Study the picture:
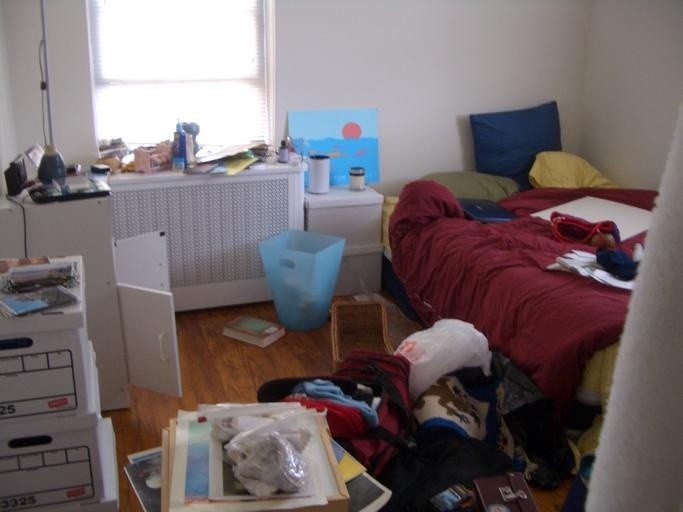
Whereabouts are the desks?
[108,162,306,314]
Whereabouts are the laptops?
[457,197,515,223]
[27,177,112,204]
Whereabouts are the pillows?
[529,152,618,188]
[422,171,520,203]
[469,99,562,192]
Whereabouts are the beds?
[383,188,660,431]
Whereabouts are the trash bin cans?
[260,230,346,332]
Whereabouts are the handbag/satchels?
[473,473,539,512]
[551,211,621,244]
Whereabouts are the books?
[0,285,79,315]
[223,314,287,349]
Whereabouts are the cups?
[308,155,330,193]
[75,159,92,180]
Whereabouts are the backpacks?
[258,349,413,480]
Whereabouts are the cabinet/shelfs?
[0,177,182,412]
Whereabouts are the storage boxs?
[0,256,120,512]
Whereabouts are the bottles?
[350,167,365,190]
[278,139,289,163]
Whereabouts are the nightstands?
[304,184,384,297]
